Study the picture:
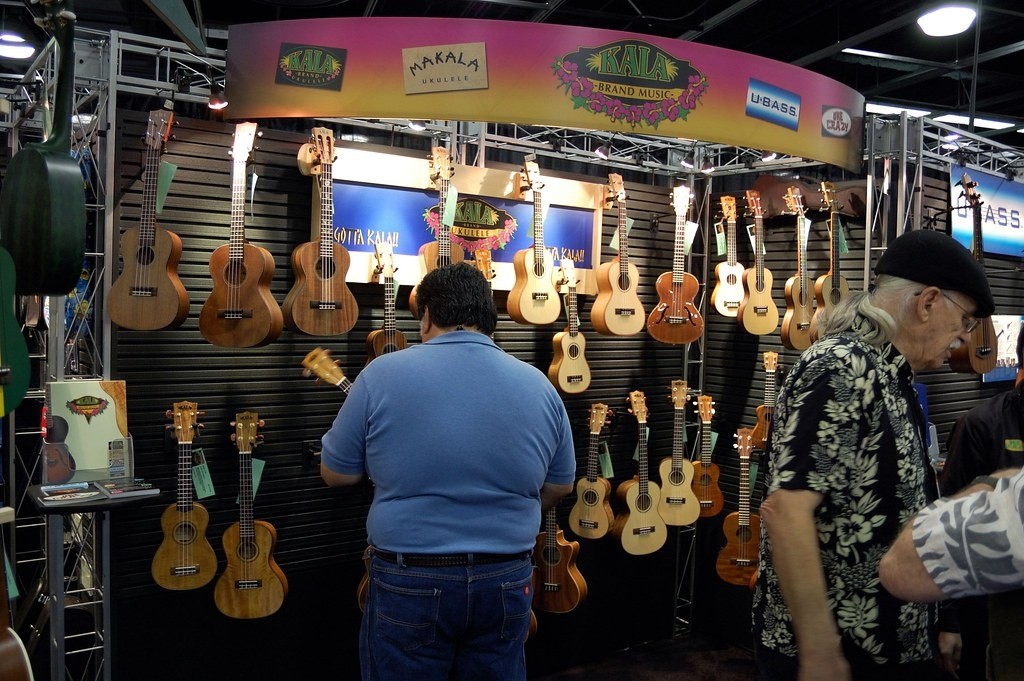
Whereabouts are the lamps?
[174,68,230,109]
[408,120,427,132]
[761,150,776,162]
[701,145,753,174]
[632,142,697,169]
[553,131,617,160]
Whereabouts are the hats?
[875,229,996,319]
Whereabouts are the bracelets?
[971,475,999,489]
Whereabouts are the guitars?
[548,258,591,394]
[469,248,496,344]
[409,145,464,320]
[647,184,704,345]
[365,242,408,366]
[708,179,850,351]
[214,409,288,619]
[507,161,561,326]
[199,121,283,348]
[301,347,354,394]
[591,173,646,337]
[150,399,217,591]
[714,426,760,586]
[532,503,588,613]
[281,126,359,336]
[107,109,191,331]
[947,172,998,374]
[569,377,724,556]
[750,352,779,451]
[0,0,87,681]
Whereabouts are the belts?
[373,548,530,569]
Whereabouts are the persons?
[937,329,1024,679]
[879,465,1024,681]
[321,260,576,681]
[751,229,995,681]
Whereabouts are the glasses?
[914,289,981,333]
